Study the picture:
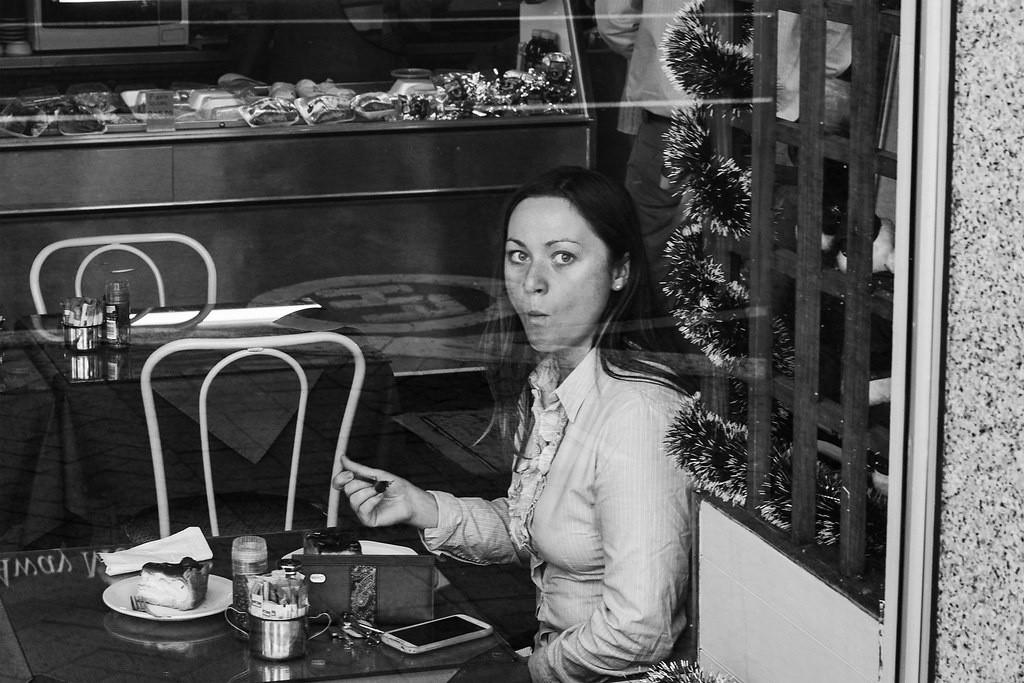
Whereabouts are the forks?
[342,468,395,494]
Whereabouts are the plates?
[237,87,445,128]
[103,574,232,622]
[0,17,28,41]
[0,92,120,138]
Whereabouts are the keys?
[329,614,383,649]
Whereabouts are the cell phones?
[381,614,494,654]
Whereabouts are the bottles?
[103,278,131,351]
[516,29,558,72]
[231,536,268,622]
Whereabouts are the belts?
[641,109,686,126]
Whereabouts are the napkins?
[98,526,214,576]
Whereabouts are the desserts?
[303,533,363,555]
[135,557,209,610]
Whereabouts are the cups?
[225,605,332,660]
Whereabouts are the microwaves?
[25,0,189,51]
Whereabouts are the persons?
[594,0,706,313]
[332,166,696,682]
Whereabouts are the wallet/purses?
[291,554,436,622]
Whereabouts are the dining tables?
[0,524,526,683]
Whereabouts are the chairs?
[139,330,366,539]
[29,231,217,315]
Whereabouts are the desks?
[14,299,410,549]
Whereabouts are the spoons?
[338,612,385,643]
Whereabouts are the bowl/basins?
[390,67,431,84]
[61,320,105,351]
[4,41,32,56]
